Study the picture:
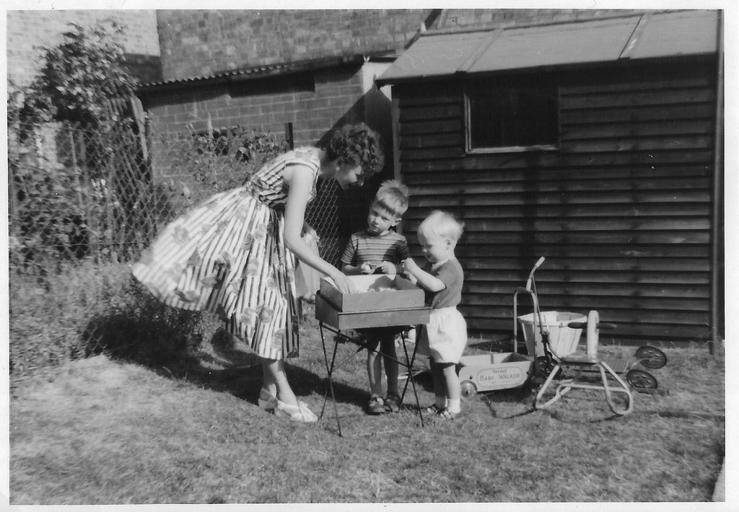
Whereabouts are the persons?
[342,179,414,415]
[129,122,384,425]
[399,209,468,421]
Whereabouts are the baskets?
[518,311,587,356]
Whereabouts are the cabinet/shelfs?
[317,321,425,436]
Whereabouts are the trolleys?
[458,256,563,402]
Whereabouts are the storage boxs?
[320,274,425,311]
[314,290,432,330]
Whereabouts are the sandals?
[422,405,444,416]
[435,413,460,425]
[384,397,398,410]
[368,396,386,414]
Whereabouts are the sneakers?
[258,390,279,411]
[271,401,316,423]
